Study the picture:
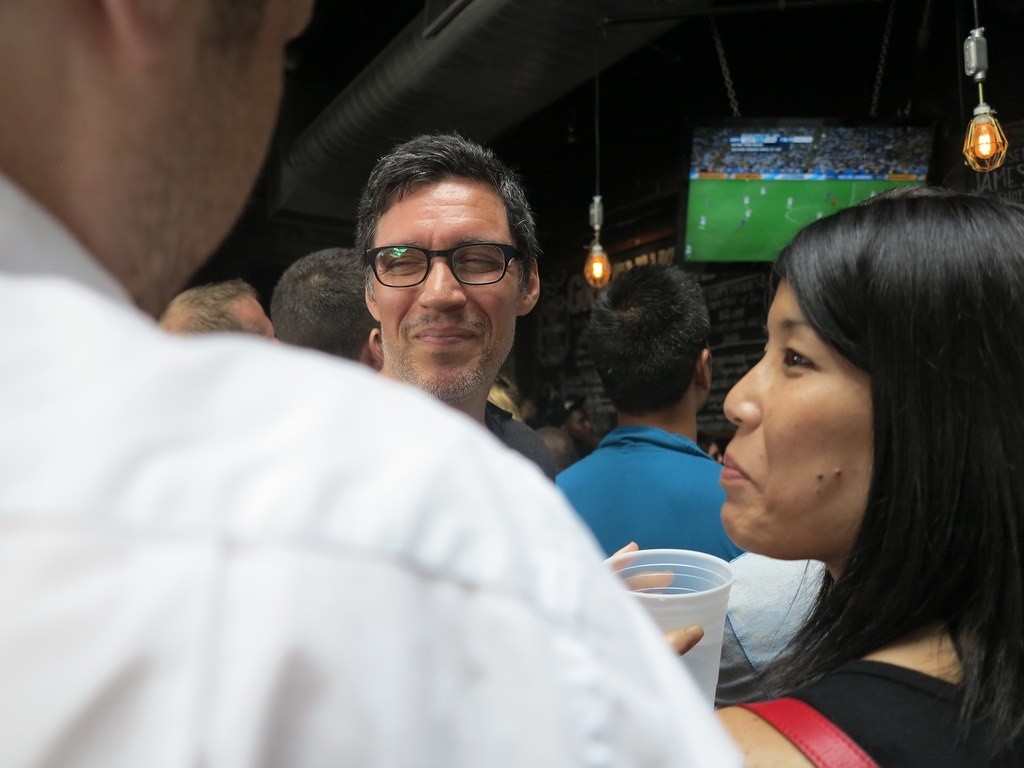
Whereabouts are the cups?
[605,549,735,718]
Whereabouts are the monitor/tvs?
[675,116,952,273]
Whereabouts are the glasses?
[364,245,523,287]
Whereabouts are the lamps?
[962,28,1009,171]
[584,196,611,288]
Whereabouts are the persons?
[0,0,1024,768]
